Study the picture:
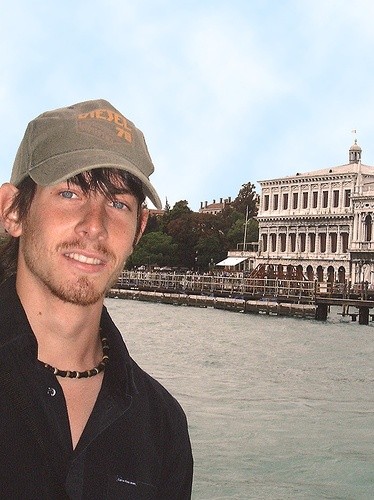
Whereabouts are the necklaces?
[37,325,110,379]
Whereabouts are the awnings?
[216,257,248,267]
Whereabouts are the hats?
[11,98,162,212]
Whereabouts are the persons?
[0,98,193,500]
